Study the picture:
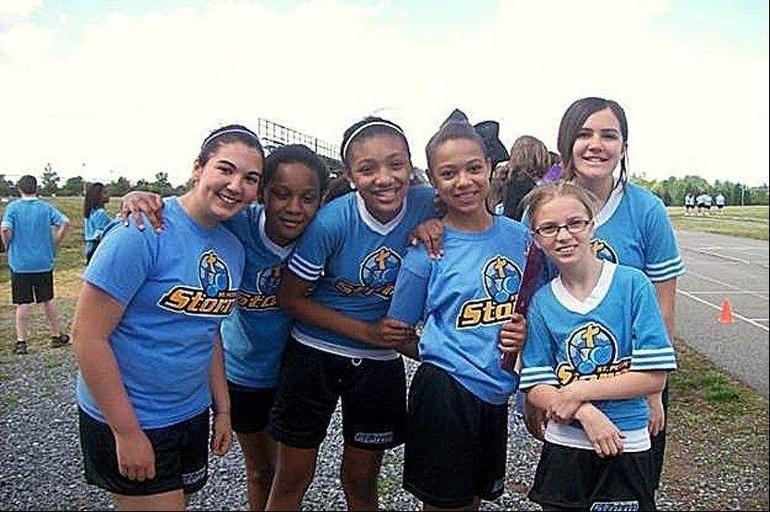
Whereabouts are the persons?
[72,97,688,512]
[682,190,726,219]
[0,174,71,354]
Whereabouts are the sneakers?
[11,340,25,354]
[50,333,69,346]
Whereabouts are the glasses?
[537,218,590,238]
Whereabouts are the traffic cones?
[714,297,737,325]
